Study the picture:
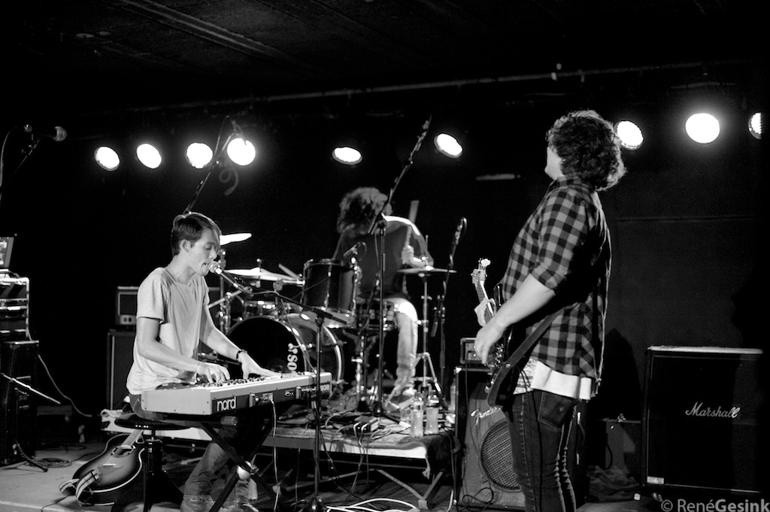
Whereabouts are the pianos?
[141,368,332,413]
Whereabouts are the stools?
[111,416,193,512]
[354,320,394,390]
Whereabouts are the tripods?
[323,330,400,431]
[347,223,407,435]
[384,280,450,411]
[282,313,345,512]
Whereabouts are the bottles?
[408,391,424,439]
[424,390,439,435]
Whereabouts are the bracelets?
[236,349,248,363]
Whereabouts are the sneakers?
[388,387,439,405]
[180,494,226,512]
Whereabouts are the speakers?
[114,285,138,330]
[1,338,41,464]
[459,382,530,511]
[641,345,764,504]
[105,329,134,411]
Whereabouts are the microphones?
[473,167,522,187]
[207,260,246,293]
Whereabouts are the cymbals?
[224,268,299,285]
[399,266,456,277]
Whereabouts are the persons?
[583,328,643,470]
[301,187,434,400]
[474,111,628,512]
[127,212,281,512]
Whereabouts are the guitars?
[71,428,148,500]
[470,256,517,403]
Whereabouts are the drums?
[301,259,362,326]
[358,298,394,332]
[225,310,342,404]
